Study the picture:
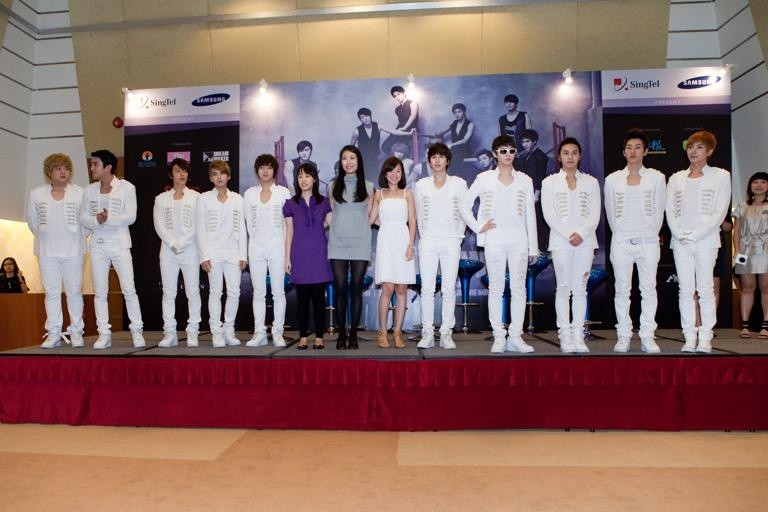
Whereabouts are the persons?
[691,203,733,338]
[457,136,539,353]
[28,154,91,349]
[323,145,380,350]
[195,160,247,348]
[415,143,469,349]
[0,257,30,293]
[283,163,333,350]
[153,158,203,347]
[666,132,731,353]
[244,154,292,346]
[732,173,768,339]
[283,85,549,251]
[604,129,666,354]
[541,138,601,353]
[80,150,146,350]
[369,157,416,348]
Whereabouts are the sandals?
[739,321,750,338]
[758,321,768,339]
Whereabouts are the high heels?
[377,331,390,348]
[393,329,405,348]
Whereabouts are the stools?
[408,274,442,340]
[267,273,295,332]
[526,250,553,334]
[481,272,510,328]
[584,250,607,340]
[375,282,410,334]
[458,259,486,334]
[345,270,373,341]
[323,284,338,335]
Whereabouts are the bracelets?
[19,280,24,284]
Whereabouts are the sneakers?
[271,333,287,347]
[70,333,84,348]
[186,332,198,347]
[439,335,456,349]
[132,333,146,348]
[157,332,178,347]
[212,332,225,348]
[560,337,590,353]
[640,336,660,353]
[92,334,111,349]
[507,337,534,352]
[491,336,507,353]
[42,335,61,348]
[680,340,697,353]
[614,336,631,352]
[224,334,241,346]
[696,338,712,353]
[245,332,268,346]
[417,334,434,349]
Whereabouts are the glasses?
[495,149,517,154]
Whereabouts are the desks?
[0,292,98,351]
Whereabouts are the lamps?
[719,63,735,77]
[407,74,414,87]
[258,79,268,93]
[122,86,148,110]
[559,68,574,96]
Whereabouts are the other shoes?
[348,336,358,350]
[336,335,347,350]
[313,344,324,349]
[297,345,309,350]
